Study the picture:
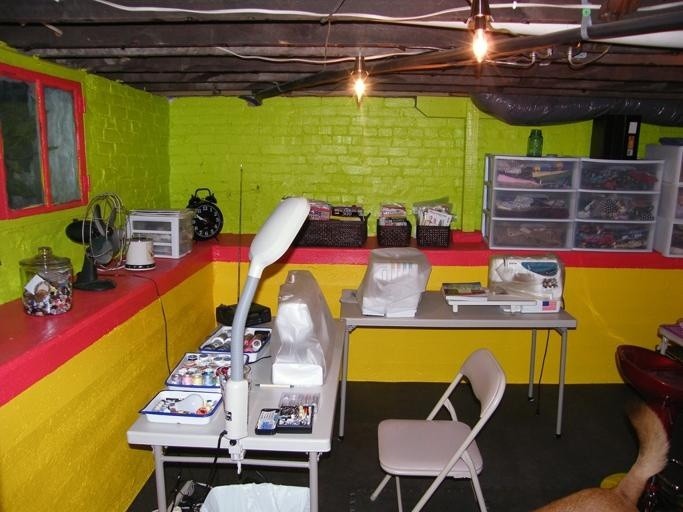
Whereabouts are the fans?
[64,193,128,292]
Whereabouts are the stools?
[600,343,682,512]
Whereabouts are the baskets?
[415,225,450,249]
[290,212,371,248]
[375,219,411,248]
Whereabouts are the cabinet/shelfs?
[478,143,683,260]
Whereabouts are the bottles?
[19,246,73,315]
[525,129,544,157]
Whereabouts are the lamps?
[223,197,310,442]
[465,0,495,66]
[349,54,369,104]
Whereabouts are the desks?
[340,291,579,438]
[125,316,347,512]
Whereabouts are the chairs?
[369,349,507,512]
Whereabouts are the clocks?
[185,188,224,243]
